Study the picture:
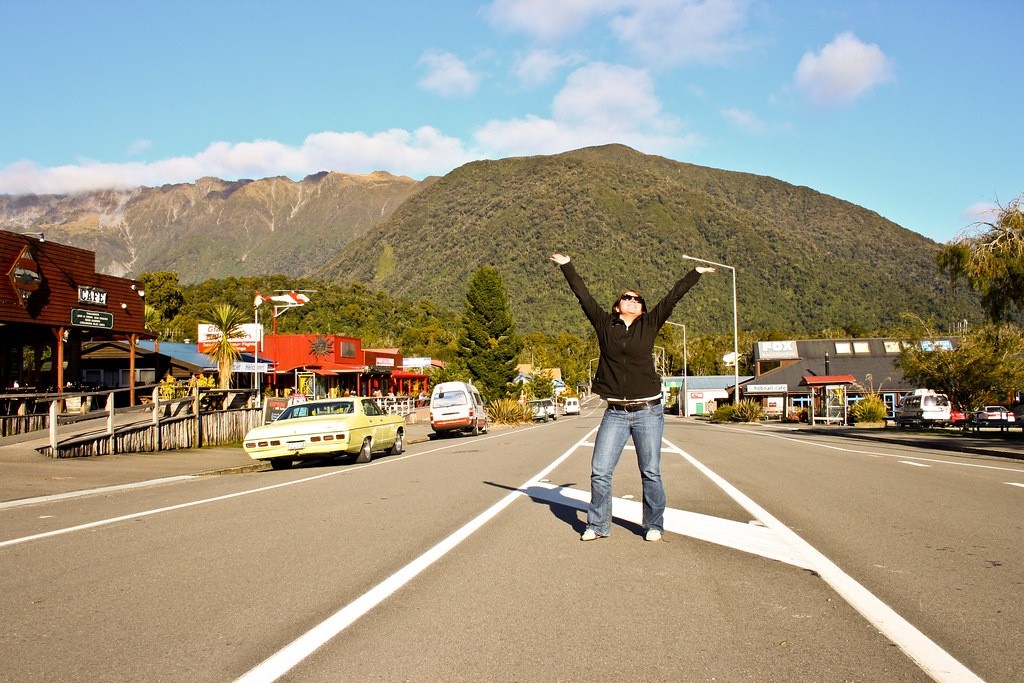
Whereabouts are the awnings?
[118,342,280,373]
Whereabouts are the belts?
[608,399,661,413]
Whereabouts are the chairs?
[0,379,102,416]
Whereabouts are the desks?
[6,386,37,414]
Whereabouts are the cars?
[526,401,548,423]
[242,398,407,470]
[973,406,1015,428]
[540,398,556,420]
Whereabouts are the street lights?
[683,254,740,407]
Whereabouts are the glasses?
[621,294,642,302]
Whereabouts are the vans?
[429,382,488,435]
[895,388,950,428]
[566,398,580,416]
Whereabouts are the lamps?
[21,231,45,243]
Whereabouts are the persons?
[548,253,715,542]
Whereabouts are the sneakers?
[646,528,661,541]
[582,528,601,541]
